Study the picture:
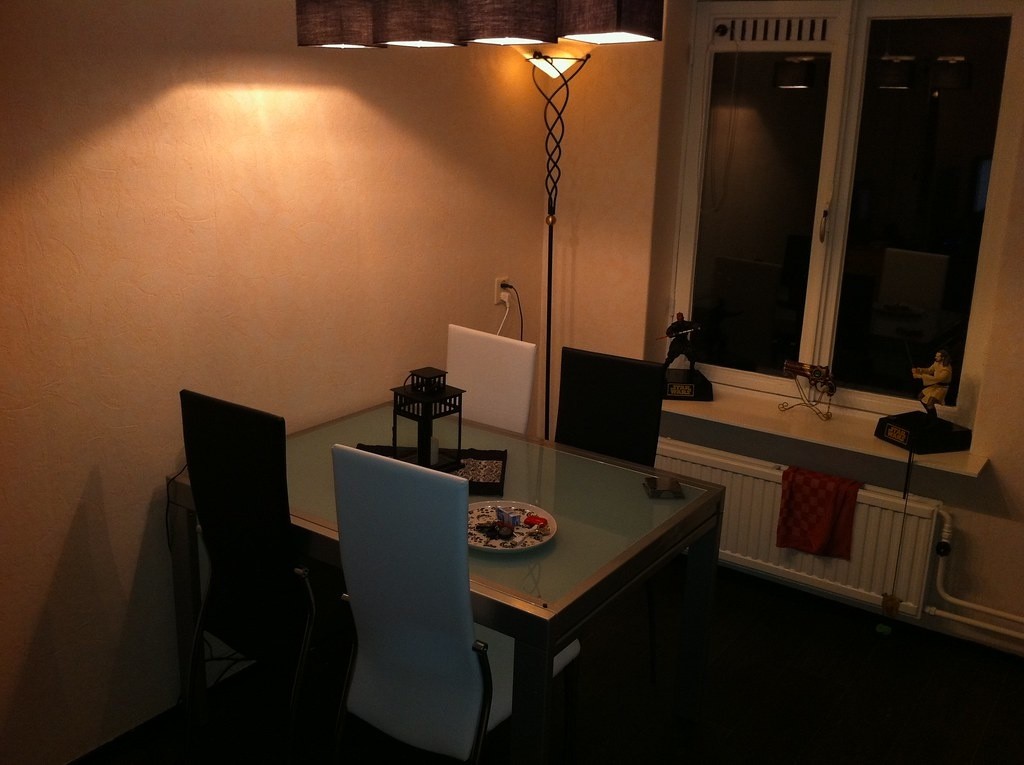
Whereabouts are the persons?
[665,312,699,372]
[911,350,953,424]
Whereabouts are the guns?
[783,358,837,396]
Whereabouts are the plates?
[466,500,557,553]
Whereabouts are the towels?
[775,464,866,561]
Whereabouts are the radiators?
[652,436,948,619]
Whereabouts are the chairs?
[446,324,536,434]
[554,345,665,469]
[172,389,355,723]
[332,443,581,765]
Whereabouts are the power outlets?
[495,277,509,304]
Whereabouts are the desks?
[165,400,726,765]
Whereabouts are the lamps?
[295,0,388,48]
[558,0,664,45]
[372,0,468,47]
[511,39,597,442]
[455,0,558,46]
